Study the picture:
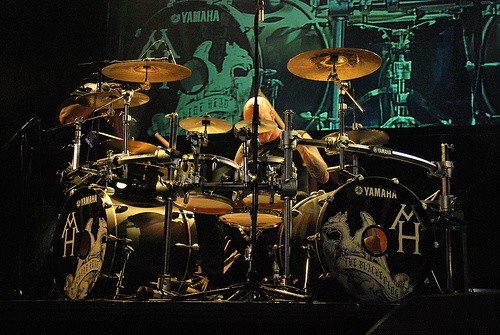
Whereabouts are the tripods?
[340,32,455,127]
[175,0,315,302]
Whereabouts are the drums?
[218,209,285,286]
[172,153,240,216]
[274,175,437,304]
[48,183,199,301]
[240,155,299,211]
[105,155,163,205]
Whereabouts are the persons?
[232,97,330,194]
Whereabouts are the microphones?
[2,117,35,152]
[259,0,265,21]
[164,42,176,64]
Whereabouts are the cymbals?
[74,90,151,111]
[100,139,161,154]
[234,118,278,134]
[178,116,233,135]
[100,60,193,84]
[322,128,391,147]
[286,47,384,82]
